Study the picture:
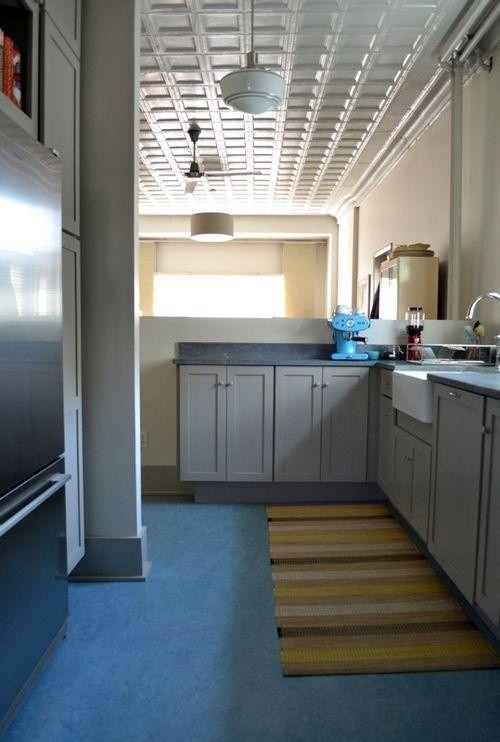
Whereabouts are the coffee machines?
[327,304,370,360]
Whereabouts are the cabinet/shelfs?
[274,365,369,484]
[378,255,439,319]
[375,367,434,545]
[177,364,274,483]
[428,381,499,631]
[0,0,40,142]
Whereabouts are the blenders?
[406,307,423,362]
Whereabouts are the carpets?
[266,501,500,678]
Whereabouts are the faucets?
[466,292,500,321]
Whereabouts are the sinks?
[392,370,483,424]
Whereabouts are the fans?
[136,124,263,198]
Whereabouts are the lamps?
[190,213,235,243]
[219,51,287,115]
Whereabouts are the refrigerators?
[0,108,72,734]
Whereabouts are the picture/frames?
[357,275,371,318]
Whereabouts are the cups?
[365,351,379,360]
[467,337,480,360]
